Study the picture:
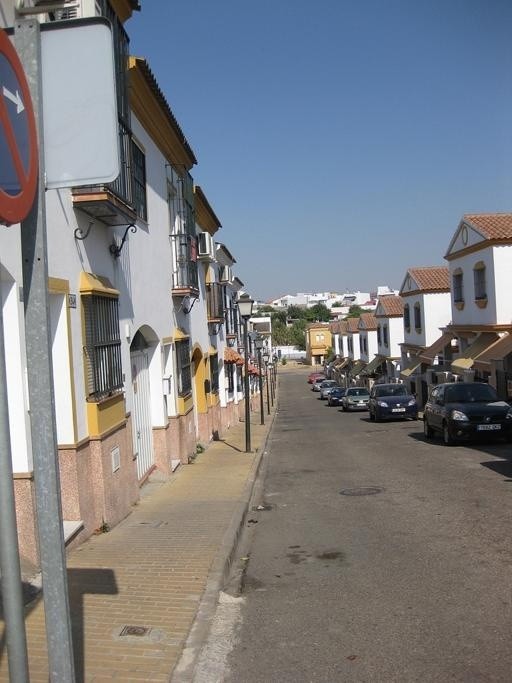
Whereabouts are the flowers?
[223,347,263,377]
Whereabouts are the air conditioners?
[198,232,233,283]
[54,0,105,22]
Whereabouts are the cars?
[308,373,371,411]
[424,381,512,444]
[368,383,418,422]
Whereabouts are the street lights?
[236,293,253,452]
[254,336,275,425]
